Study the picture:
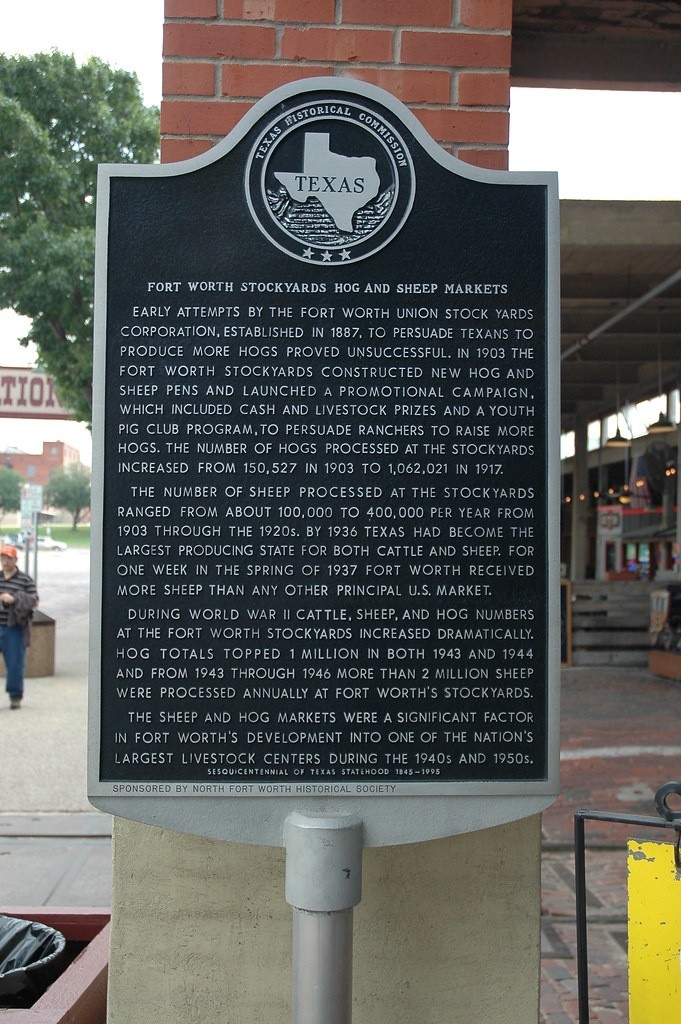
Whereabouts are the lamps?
[647,303,678,433]
[606,324,633,448]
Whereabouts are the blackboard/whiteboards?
[560,580,572,667]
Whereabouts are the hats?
[0,544,17,558]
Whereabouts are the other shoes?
[11,699,21,709]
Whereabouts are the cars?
[3,533,22,549]
[19,536,67,551]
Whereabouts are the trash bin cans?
[0,915,67,1010]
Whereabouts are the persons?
[0,544,39,710]
